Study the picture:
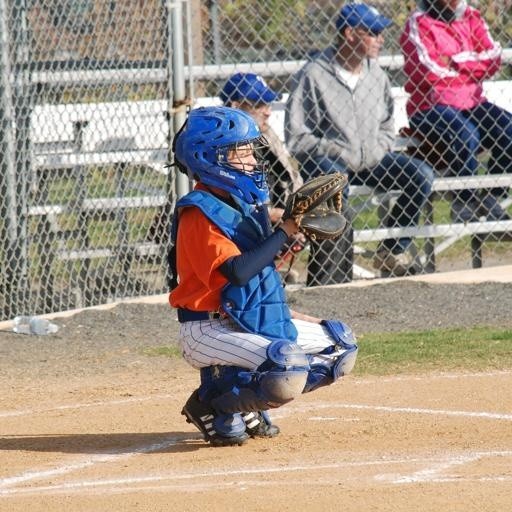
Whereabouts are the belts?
[176,308,221,323]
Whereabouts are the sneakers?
[243,410,280,440]
[182,389,249,447]
[373,252,416,275]
[477,192,510,221]
[450,201,478,223]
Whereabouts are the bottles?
[10,315,59,337]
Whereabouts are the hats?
[337,3,391,34]
[222,74,281,104]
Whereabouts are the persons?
[168,106,358,446]
[399,0,512,221]
[284,1,434,286]
[219,72,304,282]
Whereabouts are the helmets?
[175,106,272,204]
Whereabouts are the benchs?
[13,47,512,309]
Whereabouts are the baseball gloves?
[281,171,350,242]
